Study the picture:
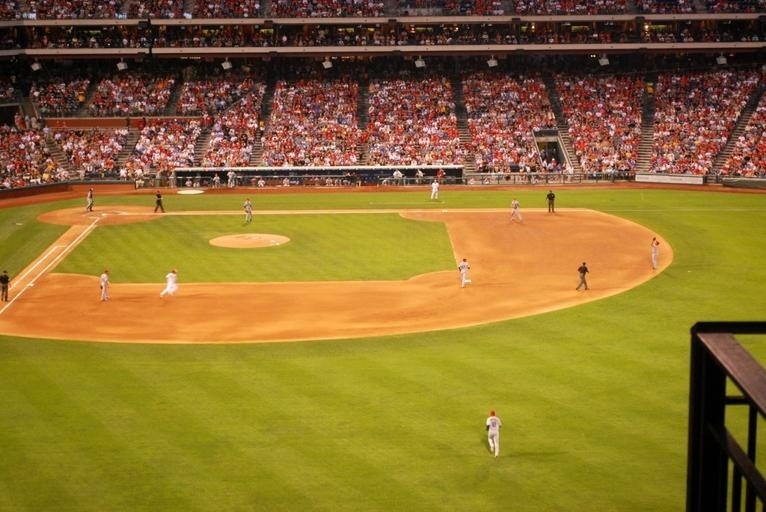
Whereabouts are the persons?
[457,258,472,289]
[152,190,166,214]
[0,270,11,303]
[429,179,442,201]
[575,262,590,290]
[483,408,503,458]
[650,236,661,271]
[158,269,178,299]
[99,270,112,302]
[545,190,555,214]
[239,198,253,226]
[0,0,766,193]
[85,187,95,212]
[509,198,525,224]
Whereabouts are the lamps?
[321,56,333,70]
[116,57,129,71]
[487,54,498,67]
[221,57,233,70]
[30,56,42,72]
[717,51,728,65]
[598,52,609,66]
[414,55,426,68]
